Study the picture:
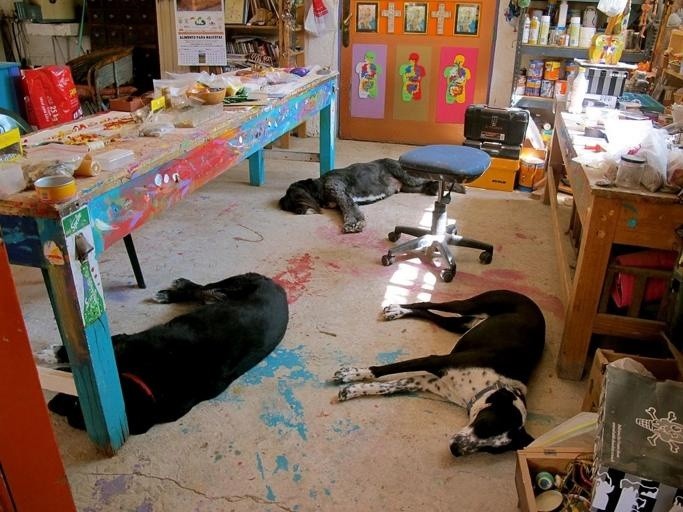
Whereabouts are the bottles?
[522,14,581,48]
[567,66,589,114]
[615,153,648,189]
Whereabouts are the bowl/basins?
[185,85,226,105]
[32,174,77,202]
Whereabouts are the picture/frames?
[355,1,481,37]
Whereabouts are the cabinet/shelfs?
[155,0,305,150]
[510,0,664,112]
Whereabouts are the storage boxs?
[462,158,522,193]
[581,348,683,427]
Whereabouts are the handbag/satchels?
[21,65,83,131]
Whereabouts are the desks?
[541,103,683,381]
[0,66,341,456]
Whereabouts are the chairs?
[0,114,146,288]
[67,44,133,112]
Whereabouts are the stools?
[381,144,494,282]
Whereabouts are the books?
[221,0,279,73]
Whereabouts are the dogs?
[33,271,289,436]
[276,157,468,234]
[331,289,546,458]
